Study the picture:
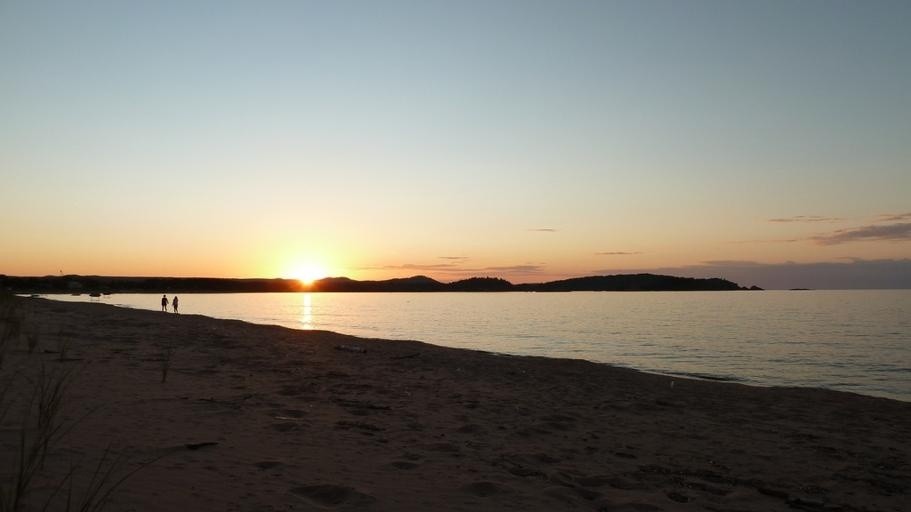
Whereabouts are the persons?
[162,295,168,312]
[173,296,178,314]
